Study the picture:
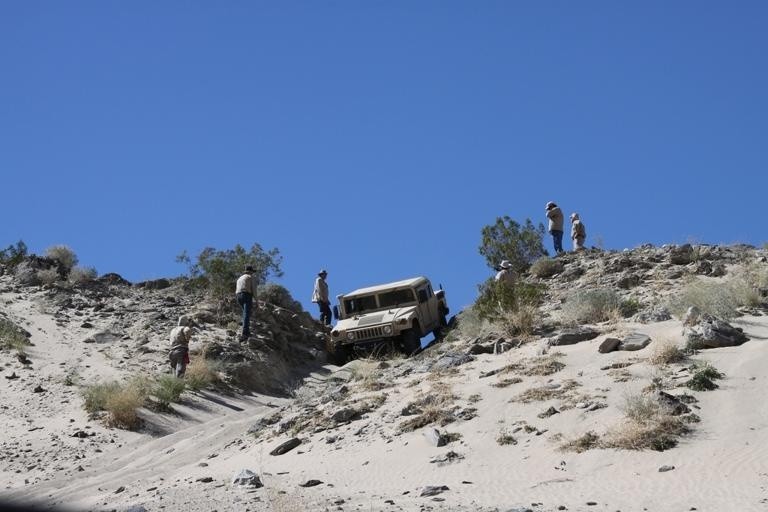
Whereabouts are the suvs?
[329,276,451,367]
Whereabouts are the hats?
[177,315,189,326]
[244,265,256,273]
[318,270,326,276]
[499,260,511,268]
[544,202,553,210]
[569,213,578,220]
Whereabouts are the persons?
[166,312,196,383]
[568,212,587,251]
[311,269,333,325]
[543,200,566,255]
[492,260,517,289]
[234,264,260,343]
